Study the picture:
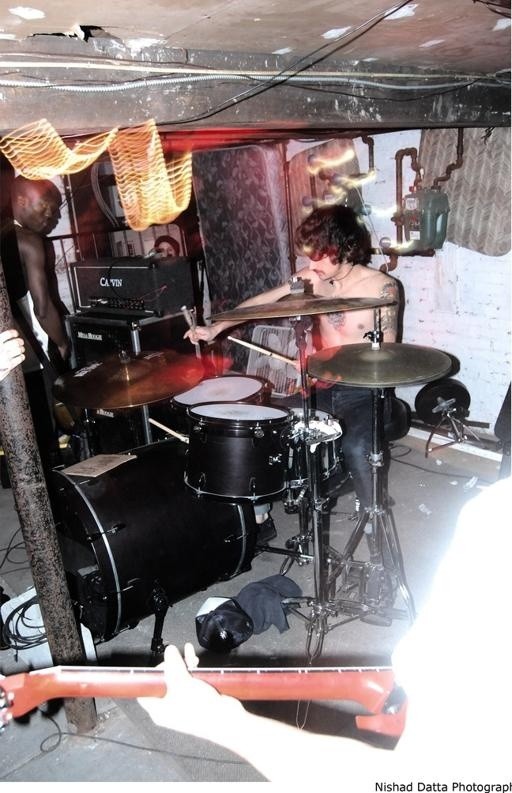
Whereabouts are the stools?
[414,378,481,459]
[348,396,412,521]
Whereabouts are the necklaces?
[327,264,355,284]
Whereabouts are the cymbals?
[52,349,205,409]
[307,343,451,388]
[206,298,393,321]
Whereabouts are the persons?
[7,173,70,365]
[132,473,511,779]
[180,203,399,517]
[151,234,213,345]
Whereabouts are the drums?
[46,434,256,643]
[284,407,345,490]
[184,402,293,506]
[170,374,272,436]
